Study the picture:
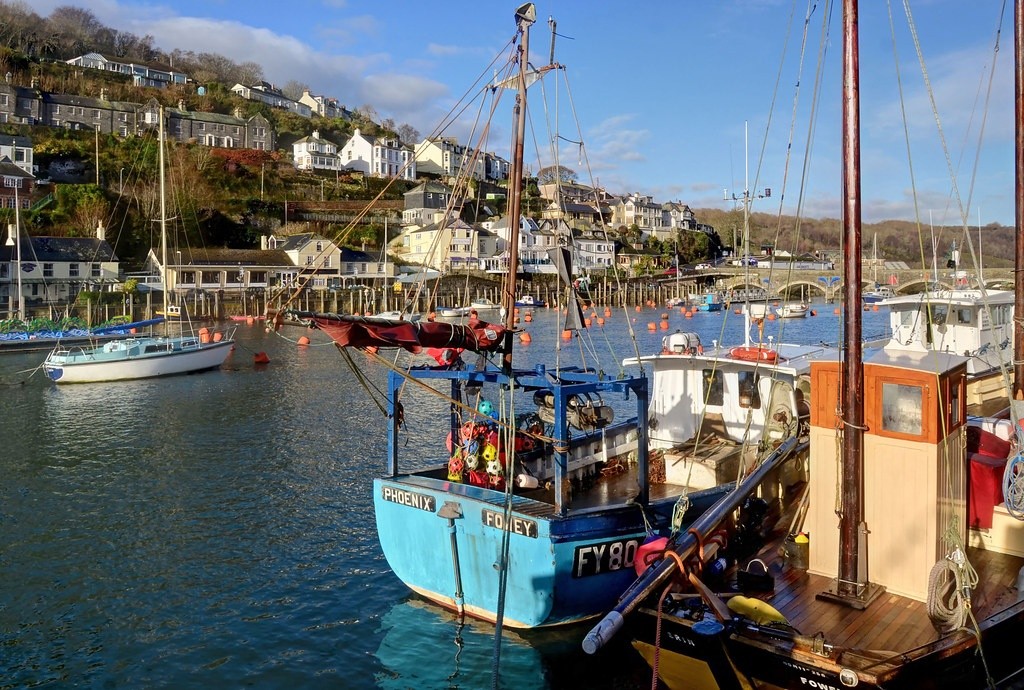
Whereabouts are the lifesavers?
[730,345,779,362]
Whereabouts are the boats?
[440,309,468,316]
[472,298,501,308]
[696,293,722,312]
[515,295,545,308]
[776,303,809,318]
[249,1,844,632]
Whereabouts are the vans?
[573,277,591,290]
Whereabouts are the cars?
[695,263,714,270]
[664,267,684,274]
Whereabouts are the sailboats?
[859,231,896,306]
[42,105,237,382]
[513,1,1024,690]
[361,217,420,321]
